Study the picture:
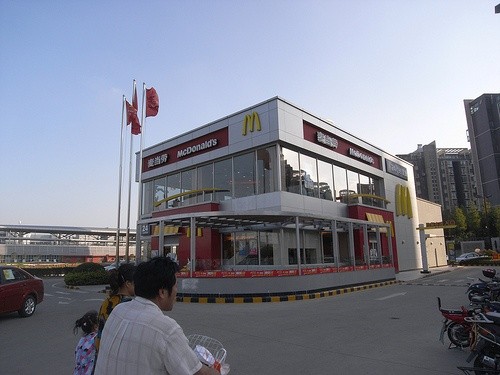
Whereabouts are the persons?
[74,309,100,375]
[91,257,220,374]
[94,261,137,350]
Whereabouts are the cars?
[104,260,135,272]
[0,266,44,318]
[456,253,485,263]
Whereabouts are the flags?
[122,80,160,134]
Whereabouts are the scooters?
[436,268,500,375]
